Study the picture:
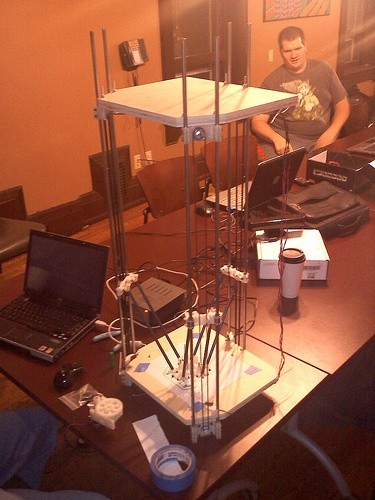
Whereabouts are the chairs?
[342,103,368,136]
[136,155,212,224]
[200,135,259,190]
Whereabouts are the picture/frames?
[263,0,331,22]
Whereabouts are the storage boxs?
[256,229,330,280]
[306,149,373,195]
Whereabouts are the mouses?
[197,204,215,216]
[55,363,84,389]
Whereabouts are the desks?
[0,217,48,261]
[0,128,375,500]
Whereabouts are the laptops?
[0,227,109,362]
[347,137,375,156]
[205,146,306,215]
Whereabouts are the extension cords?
[220,265,249,283]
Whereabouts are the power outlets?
[133,152,142,169]
[144,150,154,166]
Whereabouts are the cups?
[278,247,306,300]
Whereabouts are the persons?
[0,407,110,500]
[249,26,350,163]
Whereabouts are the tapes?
[150,445,197,493]
